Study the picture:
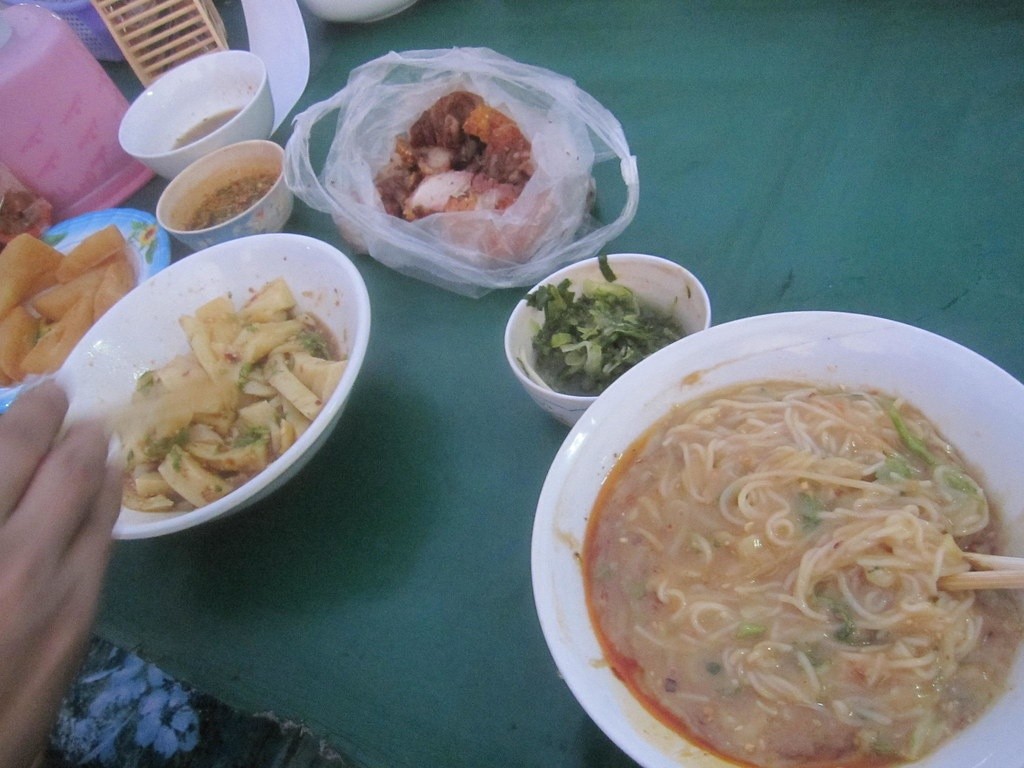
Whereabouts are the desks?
[1,1,1023,767]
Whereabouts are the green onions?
[520,256,692,397]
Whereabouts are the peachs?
[0,223,133,387]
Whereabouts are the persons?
[0,377,122,768]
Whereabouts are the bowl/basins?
[503,253,711,427]
[57,232,372,541]
[529,309,1024,768]
[155,138,293,252]
[303,0,418,24]
[118,51,276,181]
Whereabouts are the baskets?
[1,0,123,62]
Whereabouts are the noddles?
[582,388,1024,768]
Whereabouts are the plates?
[0,207,171,416]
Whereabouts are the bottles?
[0,0,157,221]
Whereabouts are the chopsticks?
[938,552,1024,593]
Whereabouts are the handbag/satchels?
[282,47,641,296]
